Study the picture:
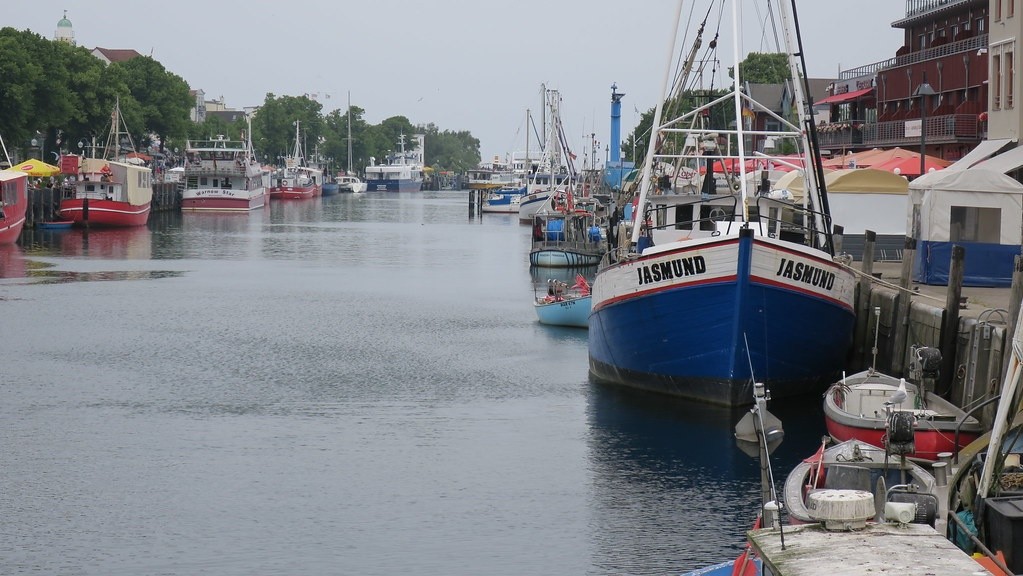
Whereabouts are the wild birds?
[883,377,907,412]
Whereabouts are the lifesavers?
[283,180,288,186]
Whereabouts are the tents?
[741,170,909,264]
[907,169,1023,284]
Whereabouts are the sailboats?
[334,90,368,193]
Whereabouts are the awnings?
[947,138,1023,176]
[814,88,873,107]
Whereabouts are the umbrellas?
[5,158,60,188]
[698,147,954,180]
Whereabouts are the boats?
[177,115,272,212]
[820,304,985,469]
[782,401,942,527]
[55,92,154,228]
[461,82,642,267]
[675,332,1015,576]
[303,137,340,198]
[0,132,30,248]
[362,129,426,193]
[584,0,860,407]
[934,293,1023,574]
[531,275,595,332]
[269,117,314,200]
[35,218,74,229]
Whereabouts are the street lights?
[55,129,65,173]
[77,137,91,159]
[31,130,45,163]
[911,68,937,175]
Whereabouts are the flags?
[743,104,755,120]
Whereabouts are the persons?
[28,175,75,190]
